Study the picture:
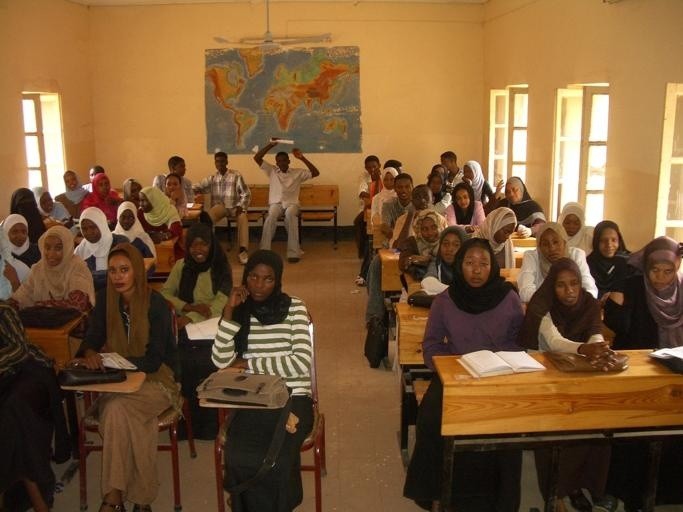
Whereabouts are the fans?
[212,0,332,47]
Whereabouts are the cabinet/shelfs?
[60,371,147,435]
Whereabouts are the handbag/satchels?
[194,369,291,411]
[54,364,128,388]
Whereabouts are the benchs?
[207,183,340,244]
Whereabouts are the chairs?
[78,301,198,512]
[214,311,325,512]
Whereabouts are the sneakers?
[239,251,249,265]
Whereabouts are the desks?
[431,349,683,512]
[498,231,538,292]
[25,305,93,363]
[144,234,178,289]
[379,248,401,371]
[396,303,431,450]
[181,203,202,226]
